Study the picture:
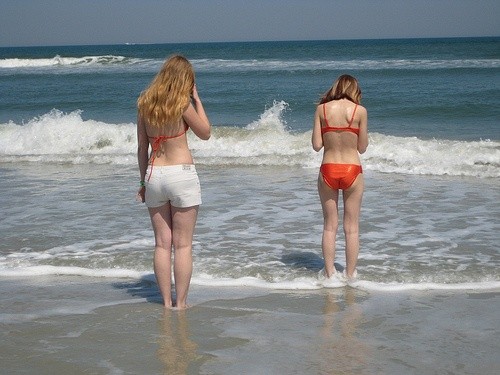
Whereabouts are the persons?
[136,55,212,308]
[311,72,369,282]
